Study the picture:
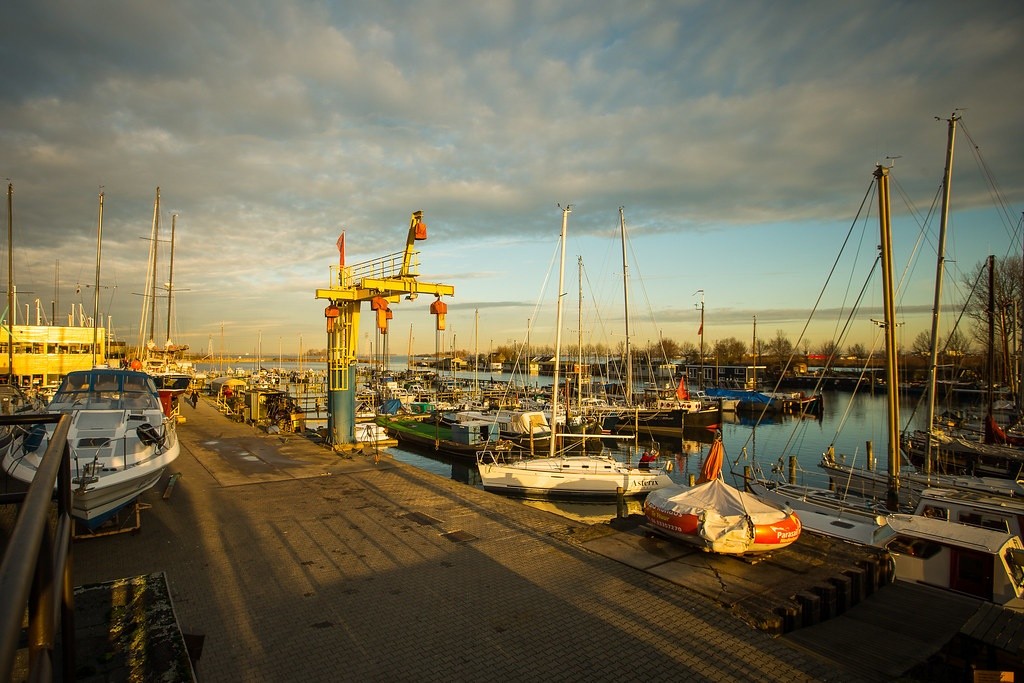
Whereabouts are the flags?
[677,380,687,399]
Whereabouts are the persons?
[172,395,178,411]
[190,389,200,408]
[639,448,659,468]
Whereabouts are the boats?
[641,477,804,554]
[0,363,182,531]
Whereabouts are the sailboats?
[2,102,1024,612]
[474,201,683,497]
